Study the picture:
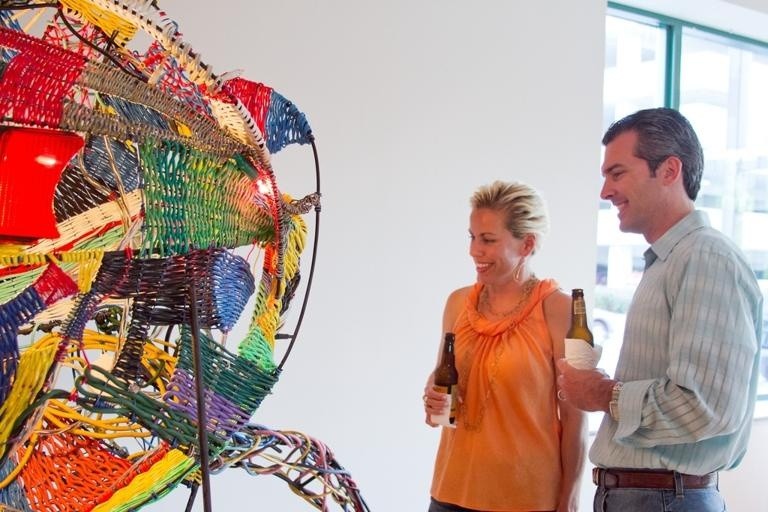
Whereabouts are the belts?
[591,467,717,490]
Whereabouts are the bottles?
[566,288,594,350]
[432,332,460,425]
